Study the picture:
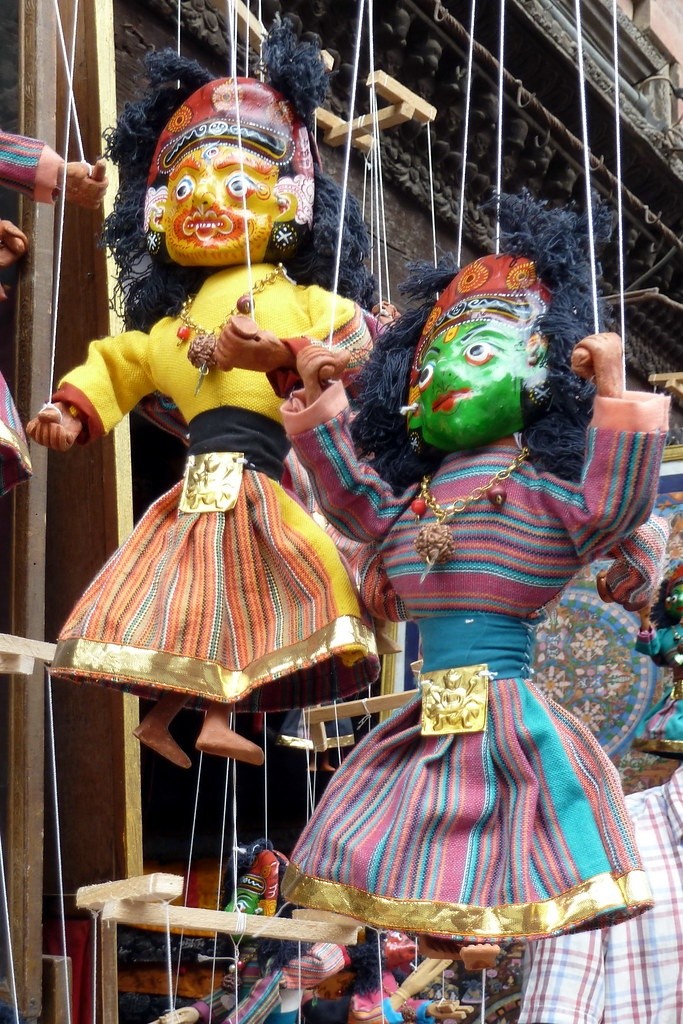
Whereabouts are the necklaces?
[404,447,532,566]
[168,262,289,370]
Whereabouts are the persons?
[348,928,475,1024]
[0,125,108,497]
[630,561,683,760]
[26,20,377,768]
[280,191,677,971]
[151,839,352,1024]
